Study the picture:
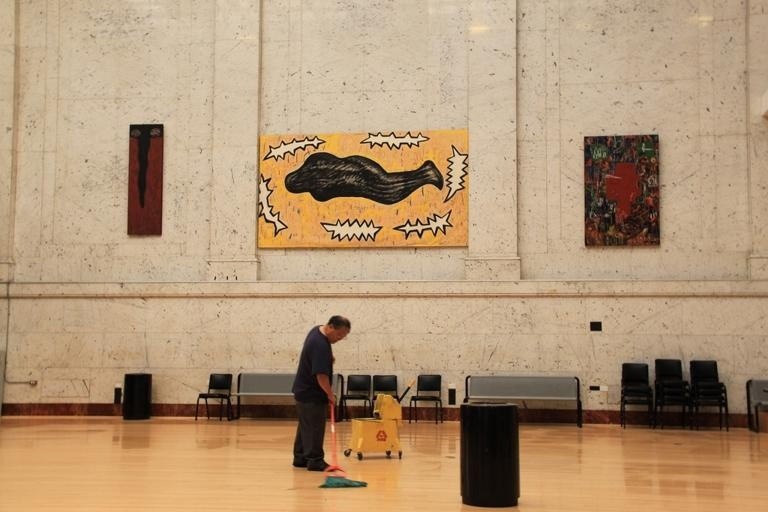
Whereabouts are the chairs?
[195,373,233,422]
[410,373,446,424]
[343,374,371,418]
[372,374,398,408]
[618,358,731,430]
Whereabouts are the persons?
[292,315,352,472]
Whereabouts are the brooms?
[316,400,368,489]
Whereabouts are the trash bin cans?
[123,374,151,418]
[460,404,521,507]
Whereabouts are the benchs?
[234,373,344,424]
[463,375,584,428]
[746,380,768,433]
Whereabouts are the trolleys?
[344,376,416,460]
